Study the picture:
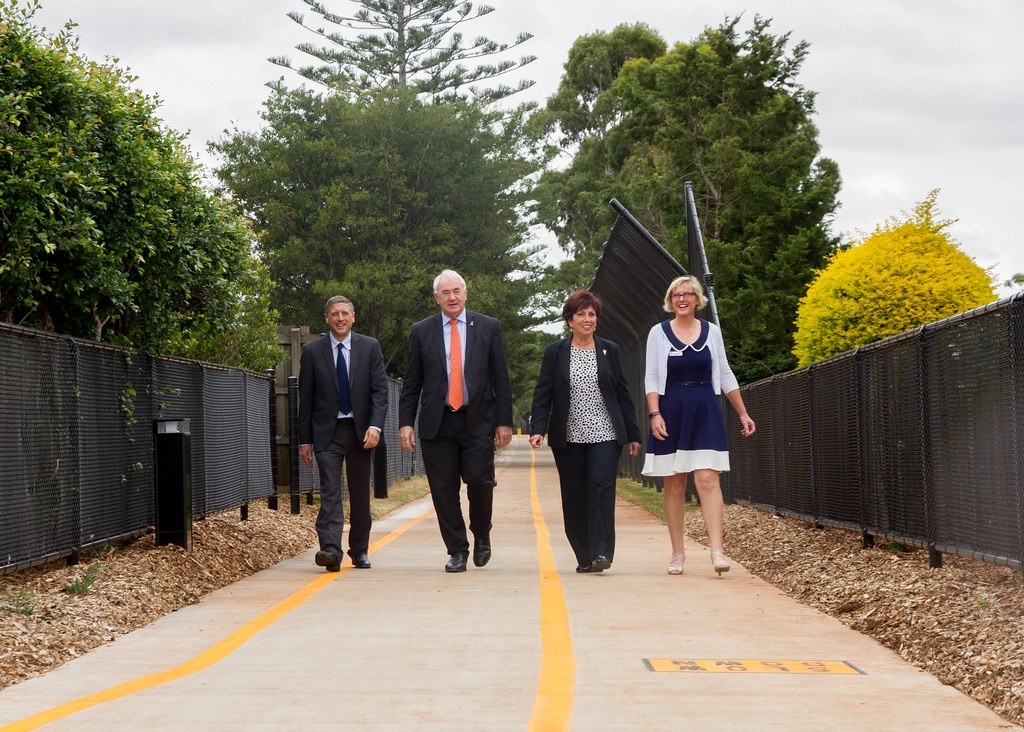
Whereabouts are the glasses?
[671,292,695,300]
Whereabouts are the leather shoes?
[576,564,591,573]
[473,531,491,567]
[315,550,340,572]
[591,554,611,569]
[445,550,469,572]
[351,552,371,568]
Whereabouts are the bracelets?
[649,411,661,419]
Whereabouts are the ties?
[448,319,464,411]
[336,342,352,416]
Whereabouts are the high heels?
[711,552,730,576]
[667,553,685,575]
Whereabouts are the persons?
[528,290,642,573]
[398,269,513,572]
[297,295,389,572]
[640,274,755,576]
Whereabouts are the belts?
[444,406,468,416]
[337,418,354,425]
